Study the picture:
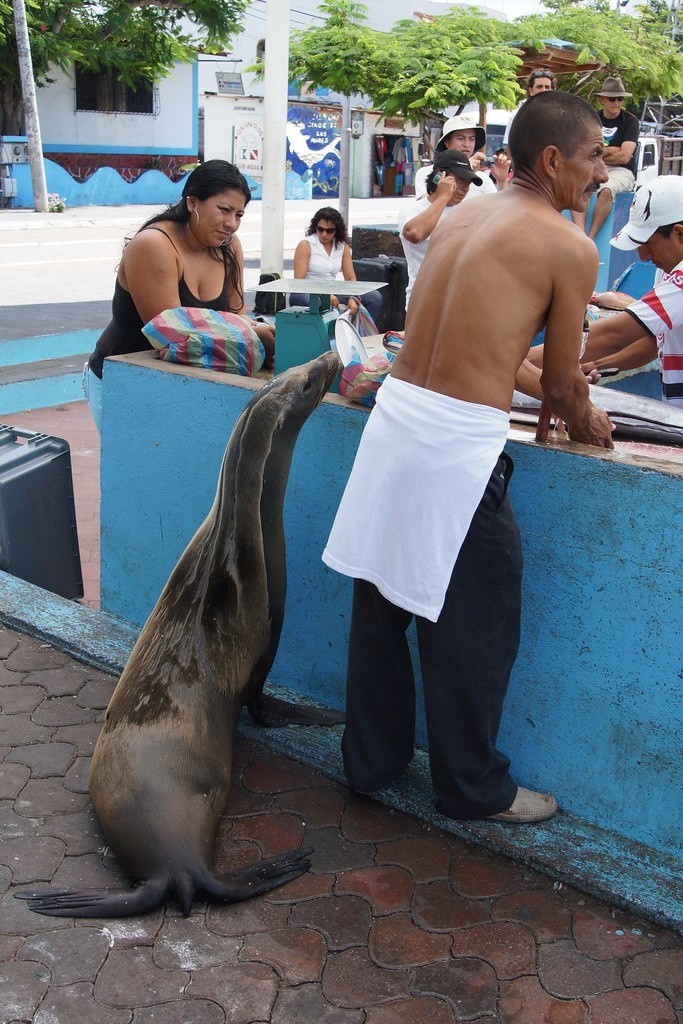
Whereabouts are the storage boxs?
[0,423,86,600]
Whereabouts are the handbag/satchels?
[339,330,403,407]
[347,296,379,337]
[141,307,266,376]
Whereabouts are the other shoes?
[484,786,558,823]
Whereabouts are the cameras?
[480,157,496,168]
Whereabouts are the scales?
[246,277,389,394]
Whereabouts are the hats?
[435,115,486,156]
[593,77,633,97]
[609,175,683,250]
[433,150,483,187]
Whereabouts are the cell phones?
[433,172,446,184]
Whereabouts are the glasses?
[607,97,625,102]
[316,224,336,234]
[534,71,552,77]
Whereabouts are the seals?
[14,348,343,918]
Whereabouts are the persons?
[82,158,275,435]
[398,148,482,312]
[288,206,382,323]
[318,90,617,820]
[414,116,512,202]
[502,68,557,167]
[526,173,683,407]
[571,75,640,239]
